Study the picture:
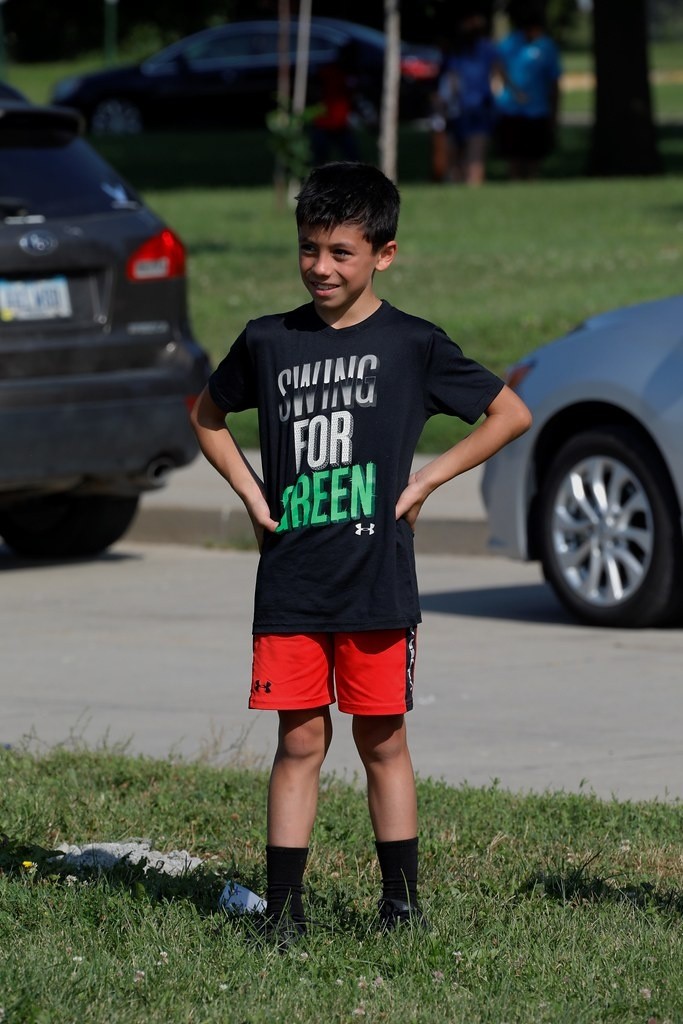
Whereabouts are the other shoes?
[267,916,304,949]
[379,900,428,936]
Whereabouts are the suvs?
[0,80,215,560]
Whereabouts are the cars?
[478,286,683,628]
[51,18,444,138]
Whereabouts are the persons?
[308,0,562,183]
[188,162,532,948]
